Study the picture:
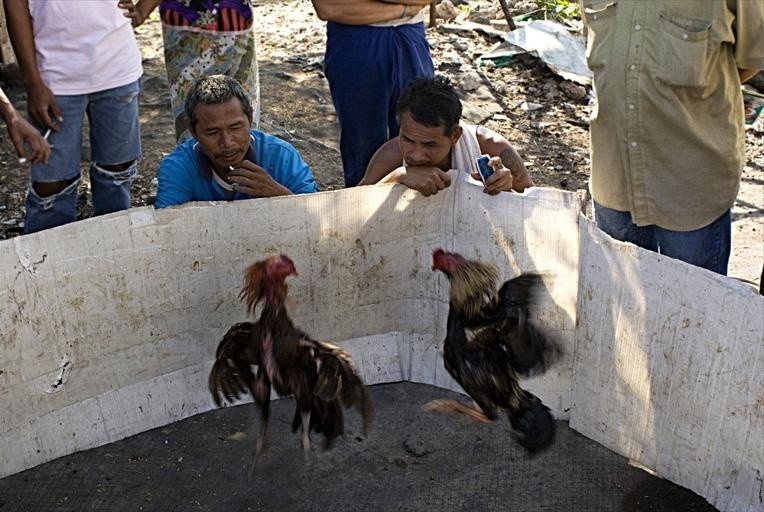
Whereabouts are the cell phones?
[476,153,496,184]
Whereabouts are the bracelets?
[401,6,409,18]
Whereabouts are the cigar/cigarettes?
[19,158,29,163]
[44,121,56,140]
[229,166,235,172]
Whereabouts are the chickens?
[420,247,564,459]
[207,252,375,482]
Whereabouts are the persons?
[155,74,319,211]
[351,74,536,201]
[3,0,161,234]
[160,0,260,143]
[580,0,764,278]
[0,83,54,169]
[311,0,437,185]
